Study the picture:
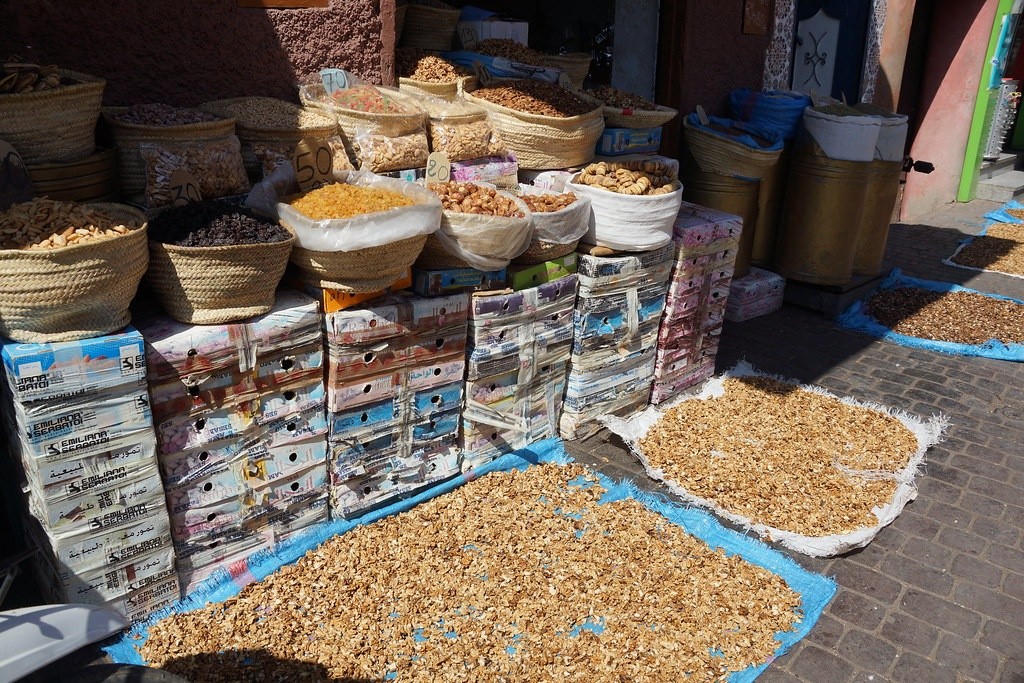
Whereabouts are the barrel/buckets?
[753,144,792,263]
[680,170,761,277]
[780,153,873,285]
[854,157,904,275]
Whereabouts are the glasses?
[1001,90,1021,132]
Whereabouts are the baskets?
[547,49,592,89]
[394,3,409,47]
[494,180,592,263]
[197,95,338,168]
[0,198,150,344]
[299,80,425,163]
[683,111,785,181]
[0,60,106,163]
[276,174,426,294]
[146,214,296,326]
[97,102,237,200]
[400,3,461,51]
[394,74,478,103]
[456,72,606,170]
[427,183,534,268]
[426,103,488,126]
[603,100,678,130]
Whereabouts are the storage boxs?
[0,201,744,625]
[595,127,662,156]
[725,266,784,305]
[724,295,783,322]
